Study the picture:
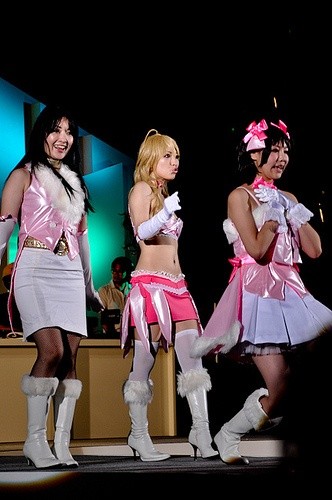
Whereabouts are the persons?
[97,256,136,339]
[210,119,322,466]
[122,128,220,461]
[0,262,23,338]
[0,100,104,468]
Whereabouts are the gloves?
[138,190,183,242]
[254,183,313,234]
[78,229,105,313]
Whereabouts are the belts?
[23,234,68,256]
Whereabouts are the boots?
[123,379,172,462]
[22,375,62,469]
[210,387,271,465]
[50,379,84,467]
[177,367,219,460]
